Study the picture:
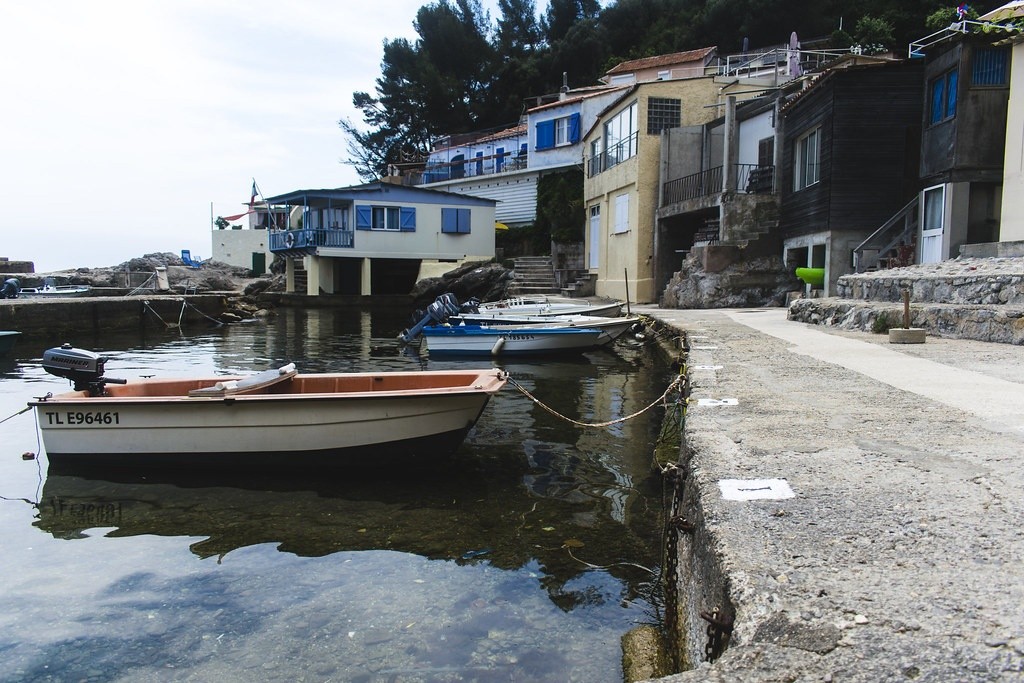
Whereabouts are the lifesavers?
[285,231,294,247]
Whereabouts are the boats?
[26,342,510,472]
[420,289,641,362]
[15,285,93,299]
